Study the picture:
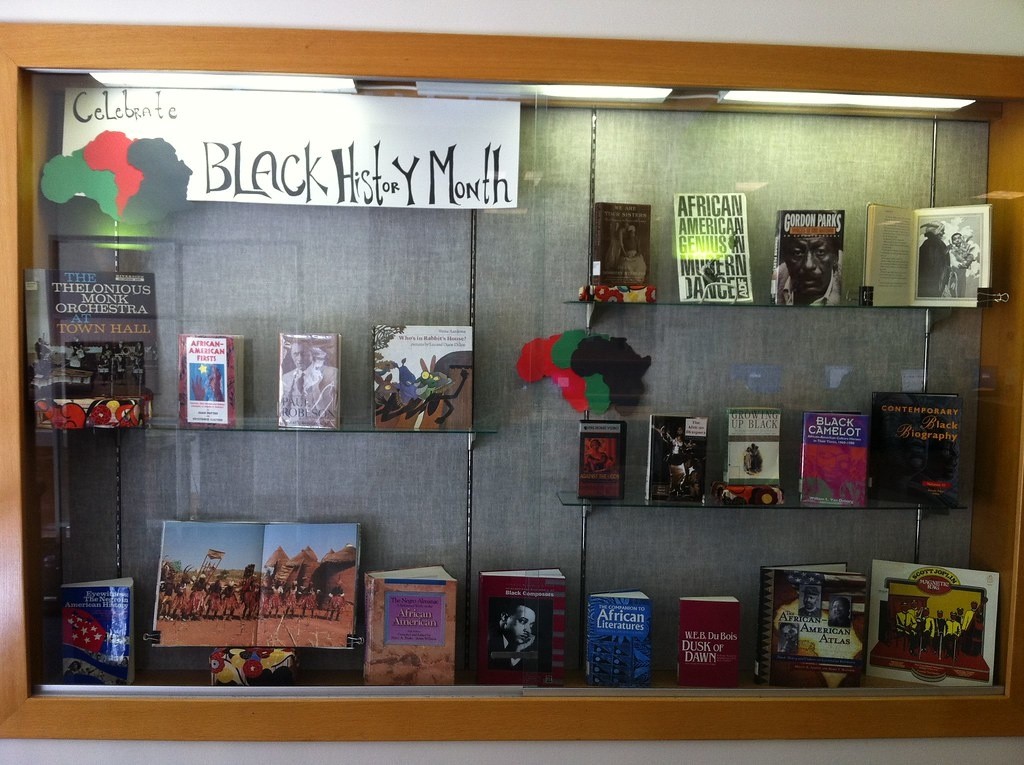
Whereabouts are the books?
[152,520,360,649]
[722,406,782,487]
[178,334,244,427]
[771,210,845,306]
[676,193,754,302]
[867,392,960,505]
[24,267,151,429]
[592,203,651,287]
[579,420,626,500]
[645,415,708,502]
[863,203,993,307]
[372,326,473,431]
[60,577,135,686]
[799,411,869,507]
[279,332,341,430]
[364,562,869,685]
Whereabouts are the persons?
[948,232,971,298]
[918,221,953,298]
[799,586,820,616]
[489,602,537,669]
[896,600,979,655]
[282,337,338,418]
[779,622,798,653]
[778,228,844,305]
[829,597,851,627]
[159,567,344,621]
[744,443,762,473]
[35,338,143,380]
